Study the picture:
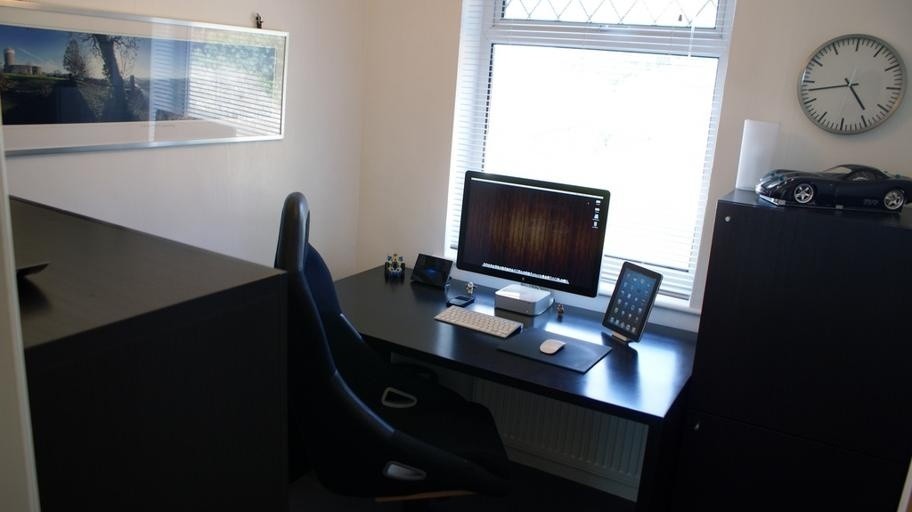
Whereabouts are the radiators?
[470,377,649,489]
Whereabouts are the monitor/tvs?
[455,170,611,317]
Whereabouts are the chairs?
[274,191,516,511]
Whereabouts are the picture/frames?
[0,1,288,156]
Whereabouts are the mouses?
[539,338,566,355]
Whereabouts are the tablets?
[602,261,664,342]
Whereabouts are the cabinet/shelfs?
[6,192,294,512]
[680,190,912,512]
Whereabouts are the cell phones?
[446,294,474,308]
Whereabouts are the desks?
[332,261,700,512]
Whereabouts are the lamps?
[735,117,779,193]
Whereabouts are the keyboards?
[432,304,522,340]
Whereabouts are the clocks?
[796,34,905,136]
